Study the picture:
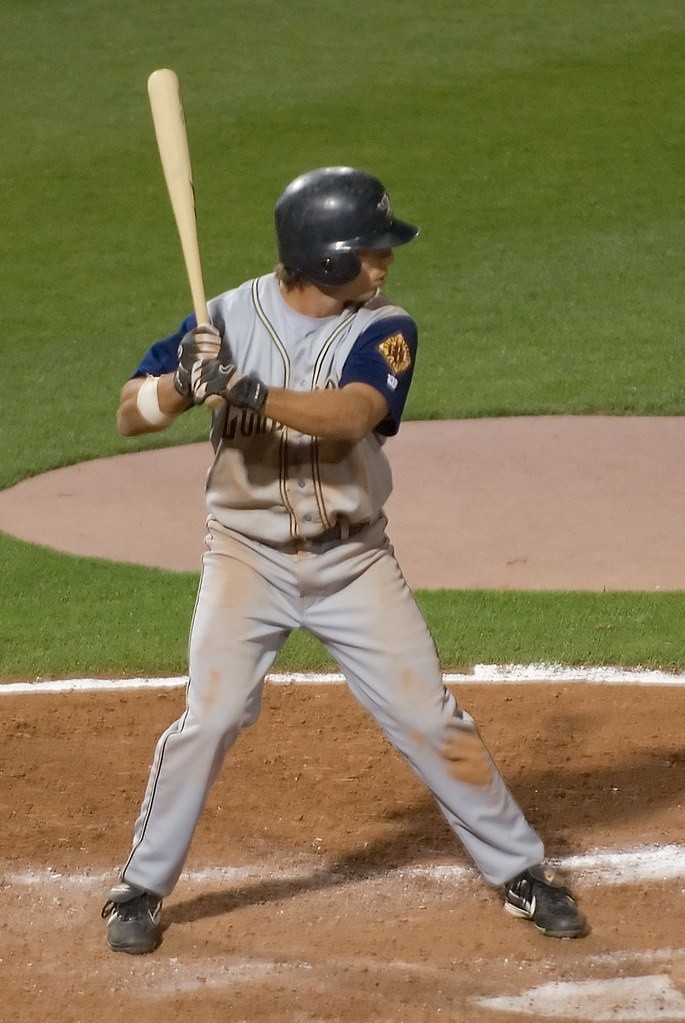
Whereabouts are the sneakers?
[502,864,584,938]
[101,880,166,955]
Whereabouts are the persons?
[101,166,591,955]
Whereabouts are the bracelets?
[136,374,176,427]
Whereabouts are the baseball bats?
[146,66,228,410]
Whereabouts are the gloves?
[173,323,268,414]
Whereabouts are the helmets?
[275,165,421,285]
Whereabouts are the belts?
[293,521,366,545]
